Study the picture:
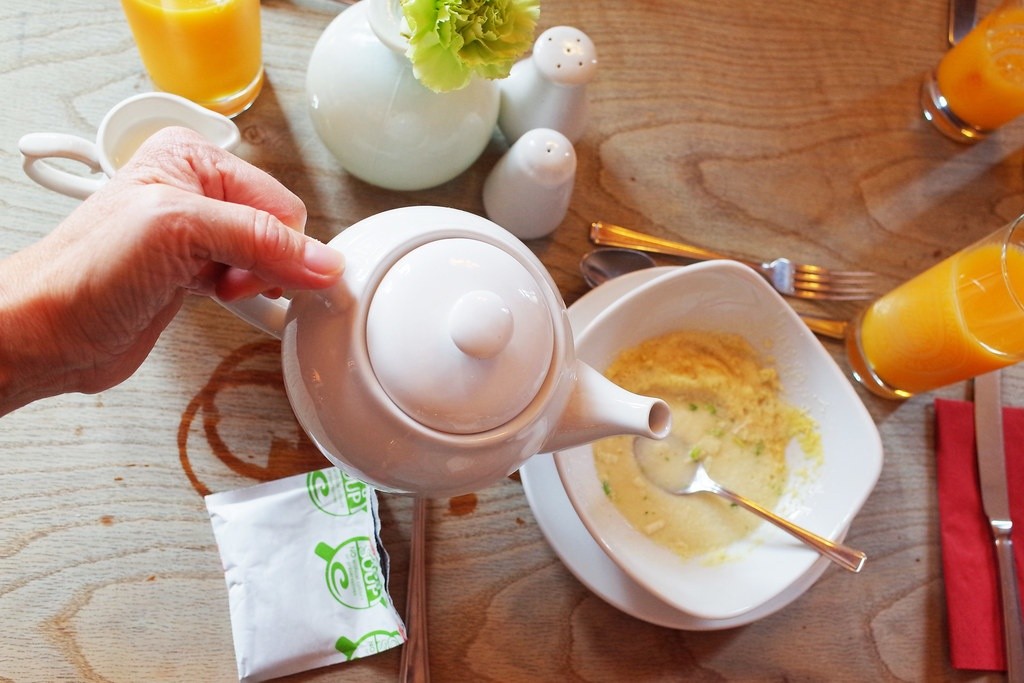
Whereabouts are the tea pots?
[212,205,675,499]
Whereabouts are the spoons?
[633,433,868,574]
[578,248,849,339]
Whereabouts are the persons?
[0,125,344,422]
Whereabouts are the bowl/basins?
[553,259,885,620]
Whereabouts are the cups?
[120,0,263,119]
[843,211,1024,400]
[919,0,1024,144]
[18,89,244,201]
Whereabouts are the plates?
[520,270,885,630]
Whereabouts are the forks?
[588,222,876,302]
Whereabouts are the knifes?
[973,367,1024,683]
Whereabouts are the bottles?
[483,128,577,240]
[501,24,597,147]
